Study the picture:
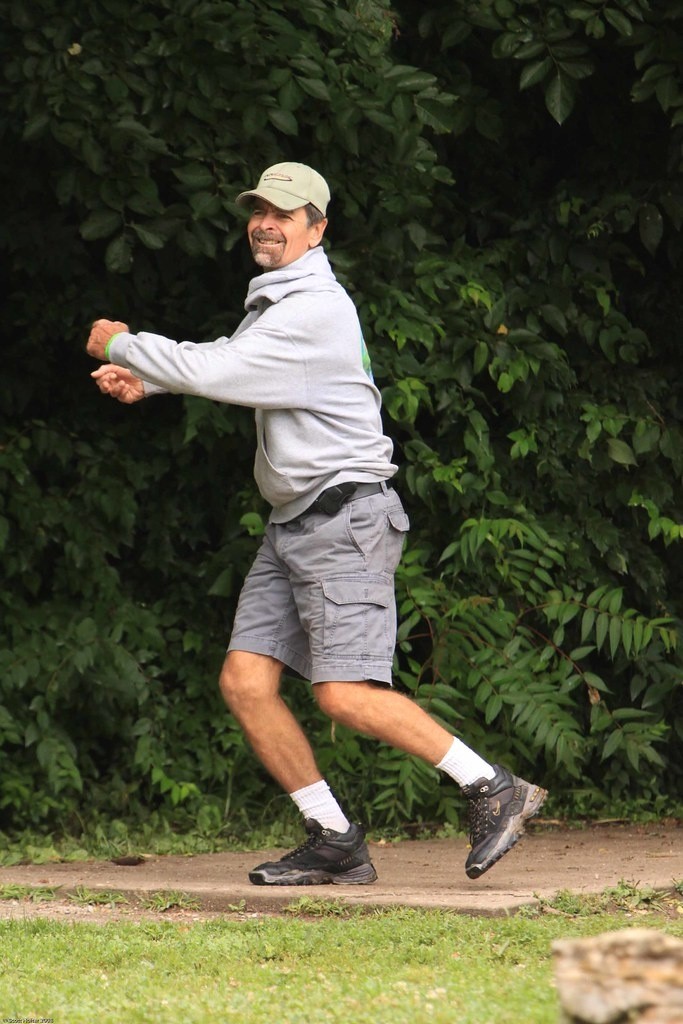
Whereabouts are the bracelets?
[105,333,120,358]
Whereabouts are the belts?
[346,478,391,503]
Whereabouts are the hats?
[235,162,330,217]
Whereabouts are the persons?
[87,162,548,885]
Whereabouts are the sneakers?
[460,764,548,879]
[250,819,378,887]
